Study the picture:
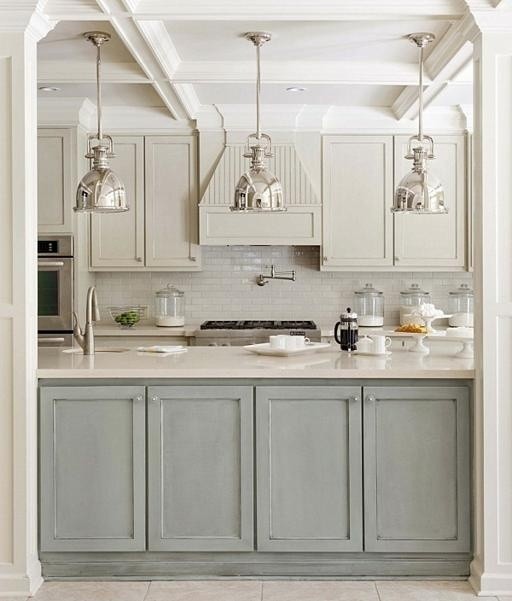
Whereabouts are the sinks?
[63,347,127,354]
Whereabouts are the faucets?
[85,285,100,326]
[259,264,295,286]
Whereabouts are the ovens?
[37,233,74,347]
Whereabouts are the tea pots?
[371,360,392,372]
[368,334,392,352]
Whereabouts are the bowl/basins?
[106,304,148,330]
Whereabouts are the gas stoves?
[194,316,322,343]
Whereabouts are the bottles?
[154,283,186,327]
[354,281,475,329]
[358,334,372,351]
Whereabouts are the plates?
[353,349,392,357]
[241,338,332,356]
[245,357,332,370]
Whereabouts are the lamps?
[229,32,288,213]
[73,31,130,214]
[391,32,448,214]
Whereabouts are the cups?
[334,307,358,350]
[268,331,310,349]
